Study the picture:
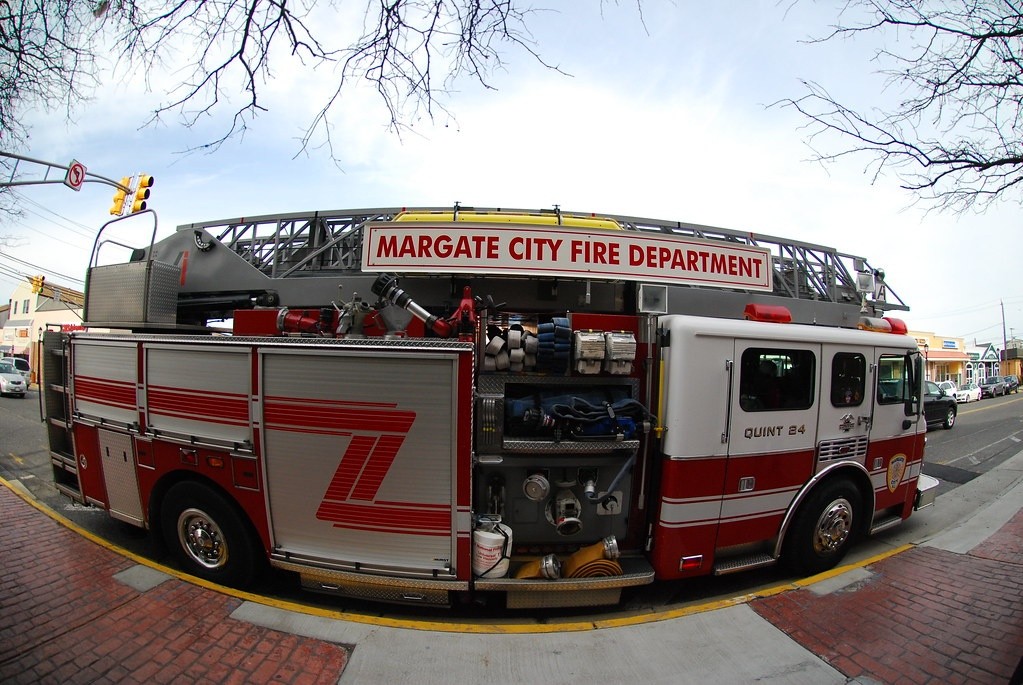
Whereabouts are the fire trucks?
[34,202,941,606]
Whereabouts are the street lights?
[923,344,932,380]
[1010,328,1015,348]
[36,327,42,383]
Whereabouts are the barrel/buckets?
[473,524,513,579]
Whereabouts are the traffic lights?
[109,176,131,215]
[32,275,45,296]
[128,174,154,212]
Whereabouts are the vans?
[1,356,31,387]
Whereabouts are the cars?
[955,384,981,403]
[0,361,28,398]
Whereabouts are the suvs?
[1004,375,1019,394]
[927,382,958,401]
[979,376,1007,398]
[880,381,957,430]
